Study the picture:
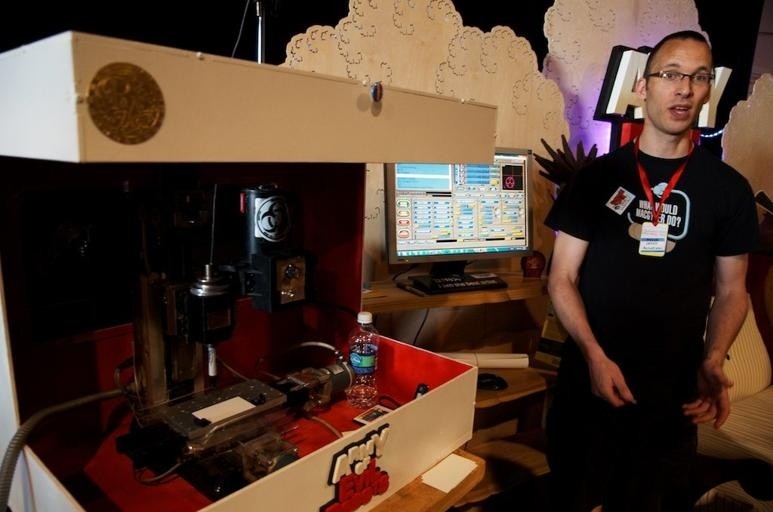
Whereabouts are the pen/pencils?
[397,282,425,297]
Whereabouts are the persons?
[543,30,760,512]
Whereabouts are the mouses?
[478,373,508,390]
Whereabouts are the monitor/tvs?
[384,147,533,280]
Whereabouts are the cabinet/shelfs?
[360,272,558,511]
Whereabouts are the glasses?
[647,70,713,84]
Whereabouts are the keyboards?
[413,271,509,296]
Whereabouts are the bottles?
[347,311,379,409]
